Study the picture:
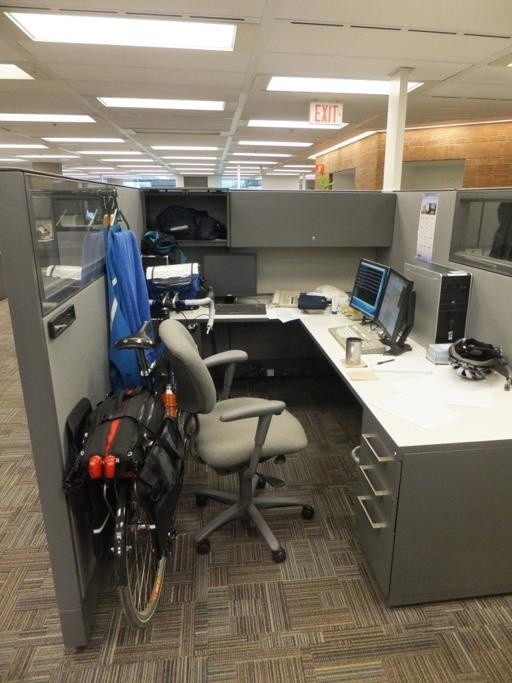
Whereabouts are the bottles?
[160,383,178,417]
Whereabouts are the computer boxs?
[403,260,472,348]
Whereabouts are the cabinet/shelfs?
[140,192,398,248]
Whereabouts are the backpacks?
[61,387,186,562]
[157,204,227,240]
[140,230,202,312]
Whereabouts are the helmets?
[448,337,503,382]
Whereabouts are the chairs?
[154,316,313,562]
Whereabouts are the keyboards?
[327,323,386,354]
[215,303,267,316]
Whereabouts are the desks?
[147,291,512,606]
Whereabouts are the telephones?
[272,290,299,308]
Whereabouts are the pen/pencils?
[378,359,394,364]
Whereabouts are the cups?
[345,337,363,365]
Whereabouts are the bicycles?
[78,291,215,628]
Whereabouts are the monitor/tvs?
[199,252,257,303]
[348,257,390,325]
[373,267,416,356]
